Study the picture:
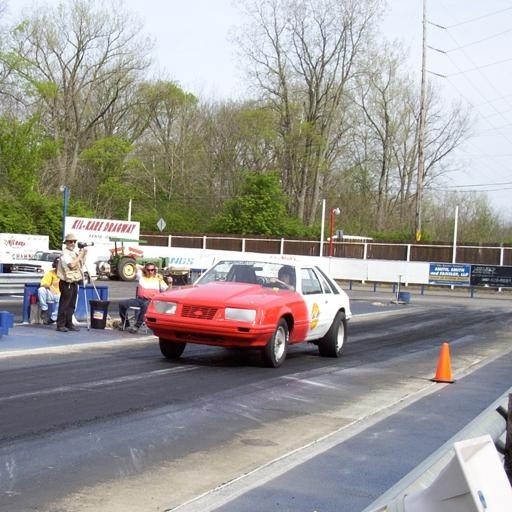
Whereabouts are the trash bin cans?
[89,300,110,329]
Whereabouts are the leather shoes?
[58,325,80,332]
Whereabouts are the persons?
[269,266,295,289]
[118,262,173,334]
[57,233,88,333]
[38,256,61,324]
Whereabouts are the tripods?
[73,256,112,331]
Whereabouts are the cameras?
[77,241,95,248]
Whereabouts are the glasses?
[147,269,154,271]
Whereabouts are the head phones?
[52,256,59,268]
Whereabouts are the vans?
[309,234,374,258]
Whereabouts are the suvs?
[11,249,90,288]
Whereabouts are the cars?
[144,258,353,368]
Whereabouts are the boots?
[40,311,54,324]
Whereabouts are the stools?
[122,306,148,336]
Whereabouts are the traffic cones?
[430,342,455,385]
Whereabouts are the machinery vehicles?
[95,236,169,283]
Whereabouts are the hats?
[66,234,77,241]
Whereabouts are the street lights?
[330,207,341,256]
[58,185,70,242]
